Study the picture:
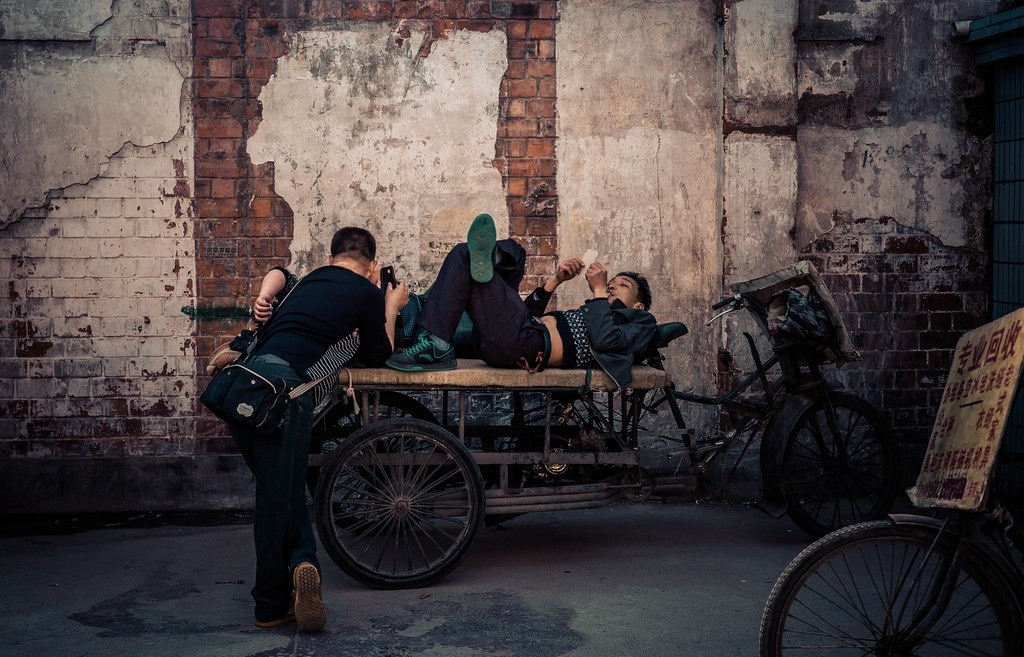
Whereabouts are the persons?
[247,267,481,360]
[225,227,409,631]
[387,214,658,388]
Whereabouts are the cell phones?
[380,266,396,295]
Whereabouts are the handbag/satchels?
[198,362,291,436]
[395,292,480,359]
[206,340,243,379]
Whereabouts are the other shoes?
[292,562,327,631]
[255,605,295,627]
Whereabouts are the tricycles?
[759,378,1023,656]
[305,281,914,589]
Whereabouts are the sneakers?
[385,330,458,371]
[467,214,496,283]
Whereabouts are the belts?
[533,316,551,372]
